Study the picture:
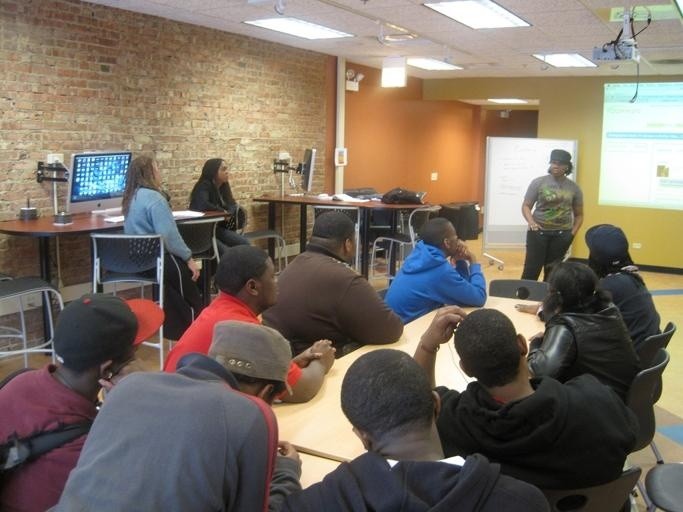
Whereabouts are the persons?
[0,287,166,512]
[526,259,640,405]
[262,210,403,359]
[163,245,336,405]
[518,148,583,299]
[412,306,639,512]
[383,217,486,325]
[189,158,250,274]
[47,320,301,512]
[280,349,550,512]
[516,223,662,355]
[120,154,201,340]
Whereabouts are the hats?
[551,149,571,163]
[53,292,167,369]
[206,319,296,397]
[585,224,628,264]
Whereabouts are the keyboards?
[104,215,124,223]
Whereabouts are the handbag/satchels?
[381,188,421,205]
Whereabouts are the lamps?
[381,55,409,91]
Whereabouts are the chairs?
[489,279,551,302]
[312,204,361,276]
[637,323,675,405]
[88,231,174,372]
[371,203,444,279]
[0,274,61,375]
[498,465,642,512]
[174,215,221,295]
[229,205,288,275]
[623,346,669,452]
[645,462,682,511]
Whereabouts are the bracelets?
[418,339,440,355]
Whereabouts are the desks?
[1,207,231,354]
[251,192,432,288]
[266,297,547,493]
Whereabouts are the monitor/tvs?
[65,149,133,214]
[300,148,316,192]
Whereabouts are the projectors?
[591,44,640,61]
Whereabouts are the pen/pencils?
[26,198,31,206]
